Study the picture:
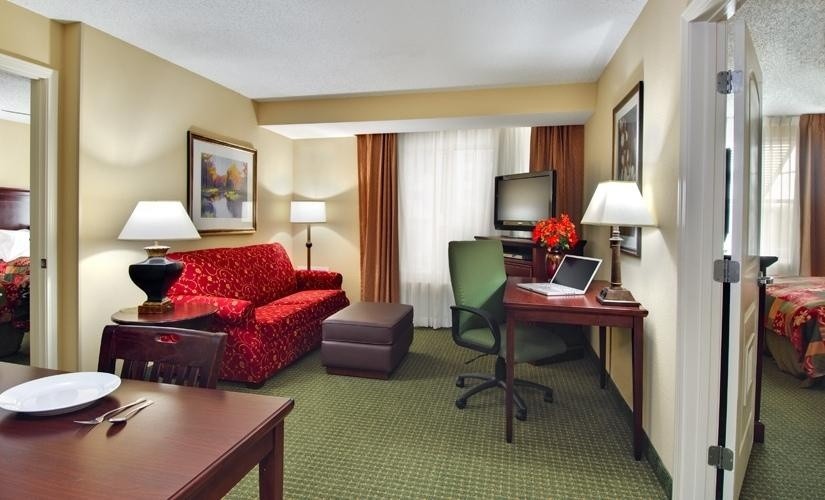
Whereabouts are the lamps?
[579,181,657,307]
[289,200,327,271]
[117,200,202,314]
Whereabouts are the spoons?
[109,401,158,424]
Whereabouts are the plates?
[0,371,122,422]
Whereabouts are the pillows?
[0,229,30,262]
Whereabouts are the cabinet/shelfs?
[474,236,587,277]
[718,255,778,443]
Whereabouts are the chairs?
[448,239,567,421]
[97,324,228,390]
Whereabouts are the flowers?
[532,210,581,250]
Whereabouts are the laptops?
[515,254,601,297]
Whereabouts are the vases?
[544,249,565,283]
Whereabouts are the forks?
[72,396,149,425]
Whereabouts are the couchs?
[165,242,350,389]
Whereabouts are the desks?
[502,276,649,461]
[111,303,219,327]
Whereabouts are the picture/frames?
[609,81,644,259]
[187,130,258,236]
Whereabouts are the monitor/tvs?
[494,169,555,238]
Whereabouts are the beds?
[764,276,825,387]
[0,187,30,333]
